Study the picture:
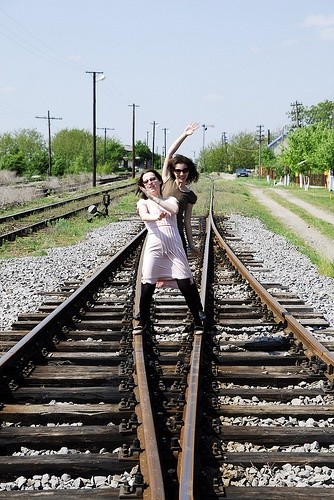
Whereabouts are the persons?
[162,120,202,263]
[131,169,205,336]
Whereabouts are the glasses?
[173,168,189,174]
[143,177,157,186]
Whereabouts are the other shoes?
[132,325,147,335]
[194,324,204,334]
[134,313,141,320]
[198,311,207,321]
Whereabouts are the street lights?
[92,74,107,188]
[202,124,208,173]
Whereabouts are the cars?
[236,168,248,177]
